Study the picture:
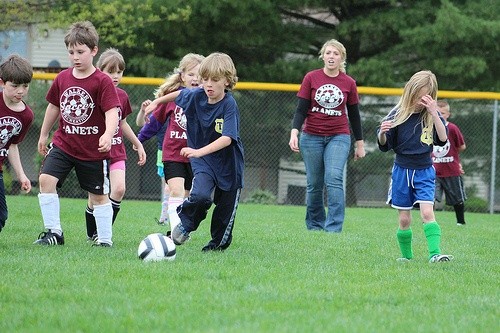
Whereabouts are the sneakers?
[88,234,99,243]
[172,222,191,245]
[34,229,64,246]
[101,243,110,247]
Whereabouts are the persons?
[85,48,146,243]
[144,52,245,251]
[377,70,454,263]
[0,54,34,233]
[288,39,366,233]
[434,101,466,225]
[136,84,186,225]
[33,20,121,248]
[133,53,205,242]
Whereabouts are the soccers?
[137,233,176,263]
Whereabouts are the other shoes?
[429,254,454,262]
[397,257,411,262]
